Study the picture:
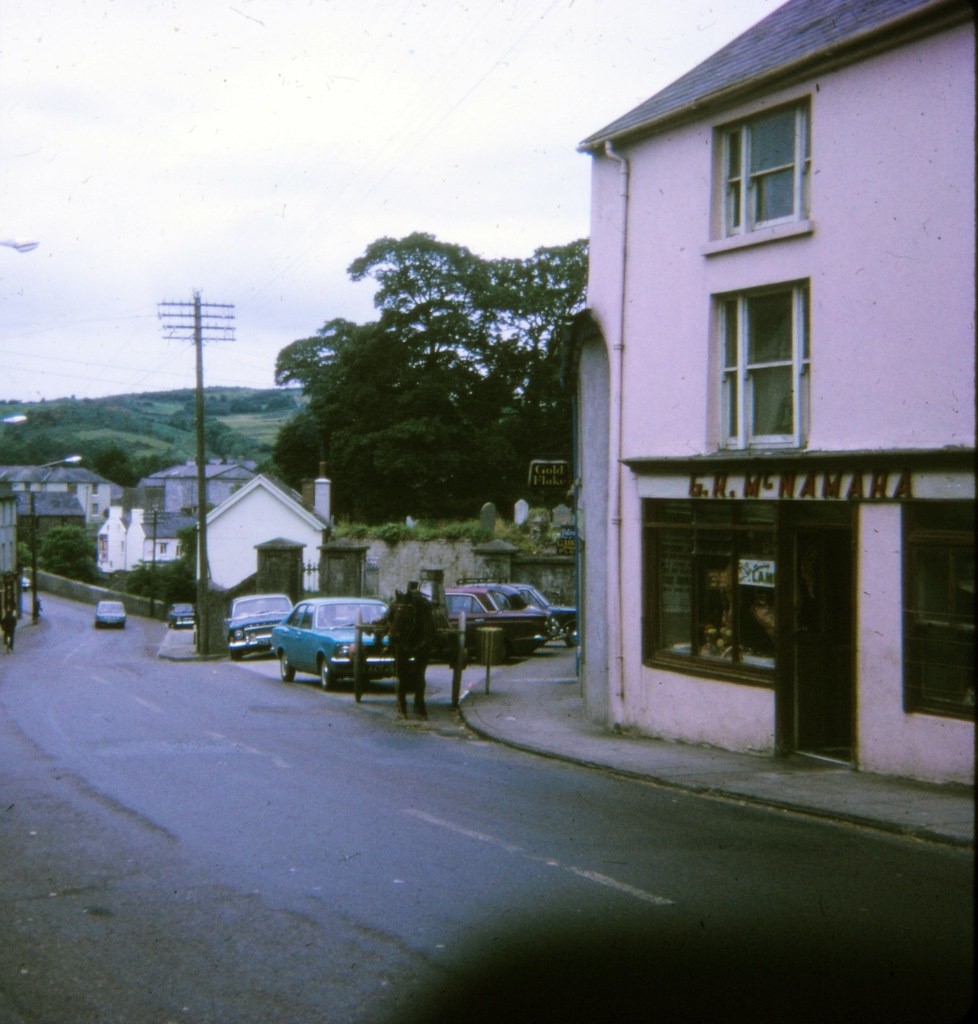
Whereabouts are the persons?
[320,606,340,628]
[361,606,374,622]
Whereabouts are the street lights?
[25,455,82,625]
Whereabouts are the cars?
[270,597,395,691]
[222,592,293,661]
[95,600,126,626]
[431,579,580,659]
[166,603,197,629]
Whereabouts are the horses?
[378,589,445,720]
